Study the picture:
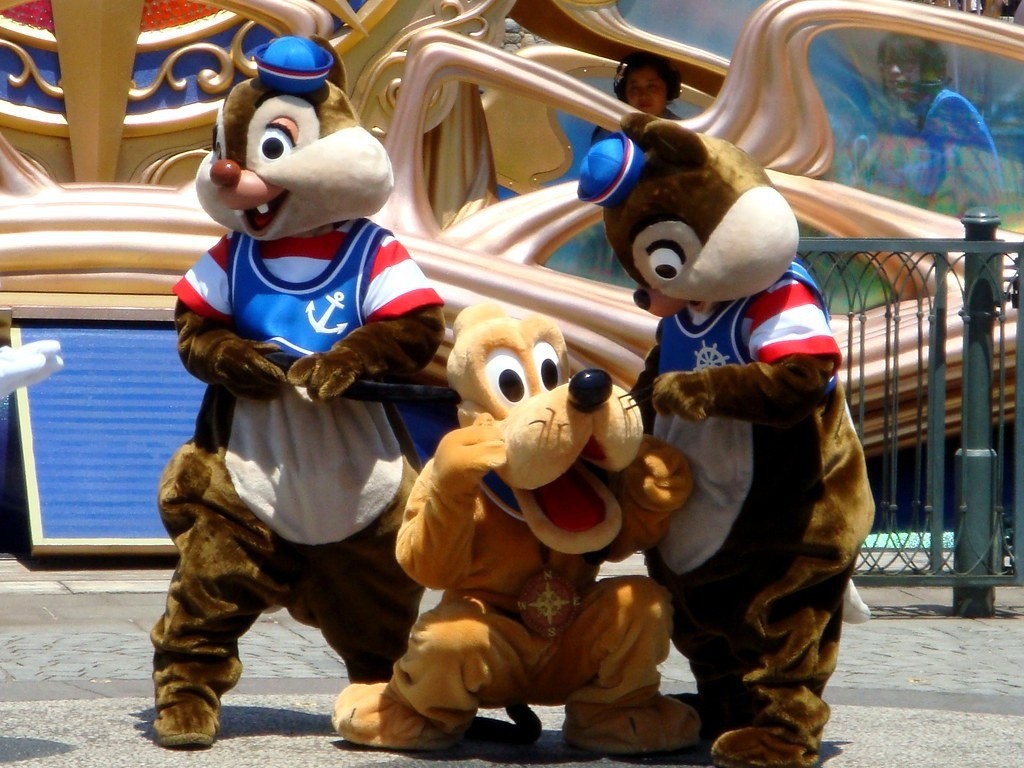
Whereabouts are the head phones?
[921,43,948,94]
[613,51,682,102]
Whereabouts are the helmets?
[613,50,681,102]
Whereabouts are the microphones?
[899,76,952,88]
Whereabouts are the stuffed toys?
[149,37,443,745]
[578,114,875,768]
[332,303,698,752]
[0,340,63,401]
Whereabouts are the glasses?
[877,54,921,72]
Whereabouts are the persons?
[578,51,684,289]
[845,31,1002,220]
